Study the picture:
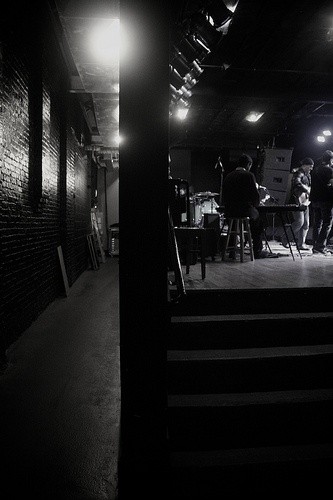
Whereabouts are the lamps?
[171,0,239,109]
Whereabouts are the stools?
[168,178,190,228]
[172,225,207,280]
[221,216,255,263]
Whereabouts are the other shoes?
[297,246,310,250]
[312,246,333,255]
[229,252,235,258]
[256,251,269,259]
[282,241,291,248]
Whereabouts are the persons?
[222,154,277,258]
[268,152,333,254]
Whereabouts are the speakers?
[258,189,288,206]
[264,149,293,171]
[260,171,293,193]
